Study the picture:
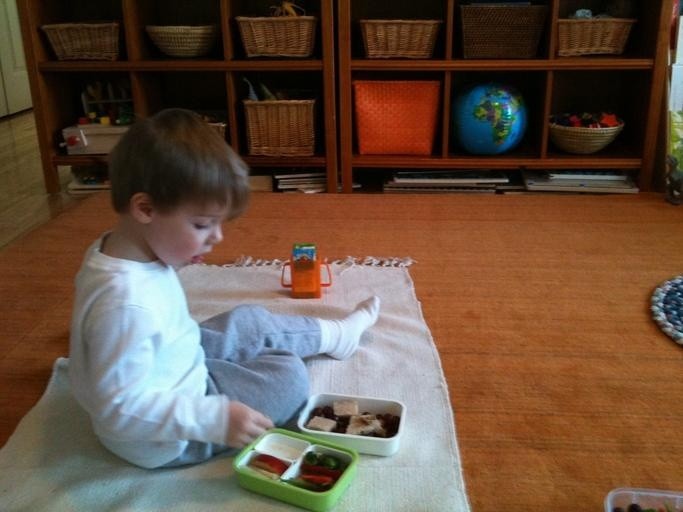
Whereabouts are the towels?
[1,262,471,512]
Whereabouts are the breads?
[307,399,381,434]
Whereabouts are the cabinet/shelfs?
[30,4,336,193]
[344,6,663,184]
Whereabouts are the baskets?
[557,19,635,58]
[550,113,625,155]
[235,16,316,59]
[144,25,217,59]
[459,3,546,58]
[359,19,442,60]
[198,109,227,141]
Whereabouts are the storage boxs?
[555,13,635,57]
[236,7,316,61]
[359,13,442,62]
[206,120,226,139]
[56,113,132,155]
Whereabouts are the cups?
[282,253,332,299]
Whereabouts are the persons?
[67,108,381,471]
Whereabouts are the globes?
[451,80,530,156]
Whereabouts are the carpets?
[649,276,683,348]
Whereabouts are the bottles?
[88,112,96,123]
